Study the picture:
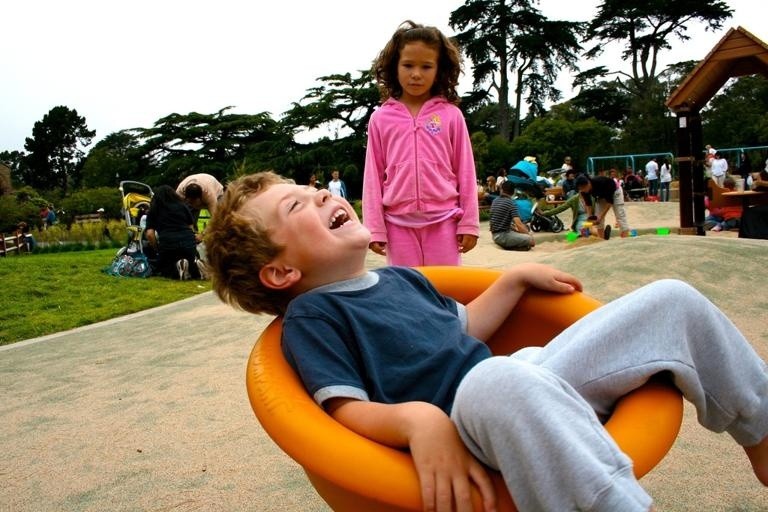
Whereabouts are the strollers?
[620,171,648,201]
[502,155,564,233]
[118,179,158,277]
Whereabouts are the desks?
[721,190,766,238]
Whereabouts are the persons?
[13,222,27,245]
[361,20,480,266]
[97,208,112,240]
[39,204,49,231]
[41,206,56,226]
[22,225,38,253]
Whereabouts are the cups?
[736,178,745,191]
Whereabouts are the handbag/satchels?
[103,255,153,278]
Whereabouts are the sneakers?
[176,259,191,280]
[193,258,209,282]
